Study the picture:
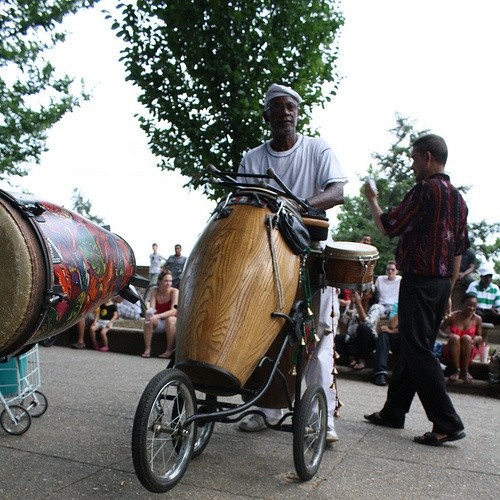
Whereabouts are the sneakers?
[239,415,280,431]
[316,428,339,441]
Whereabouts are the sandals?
[364,412,405,427]
[414,431,468,445]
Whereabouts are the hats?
[264,82,303,106]
[480,264,495,277]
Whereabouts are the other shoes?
[340,351,477,391]
[72,335,175,359]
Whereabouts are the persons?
[142,269,179,359]
[70,305,100,350]
[234,82,350,446]
[438,291,484,384]
[454,241,478,279]
[363,134,473,447]
[339,232,403,387]
[148,242,167,287]
[466,267,499,327]
[89,297,119,352]
[164,243,186,287]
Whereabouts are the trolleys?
[0,342,48,438]
[129,163,330,493]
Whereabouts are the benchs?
[73,319,500,382]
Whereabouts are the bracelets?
[299,204,306,212]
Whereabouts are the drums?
[1,186,137,366]
[171,181,312,398]
[320,238,382,292]
